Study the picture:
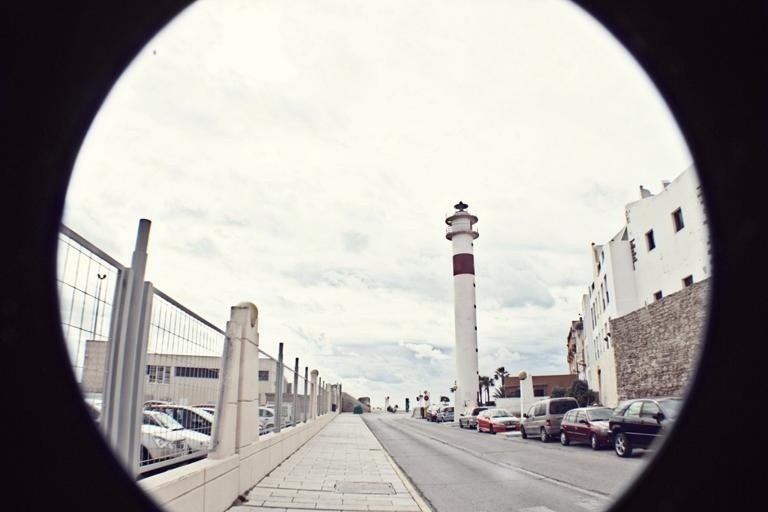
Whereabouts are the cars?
[83,398,216,467]
[259,400,294,436]
[425,396,686,457]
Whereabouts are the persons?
[410,390,431,419]
[385,396,398,413]
